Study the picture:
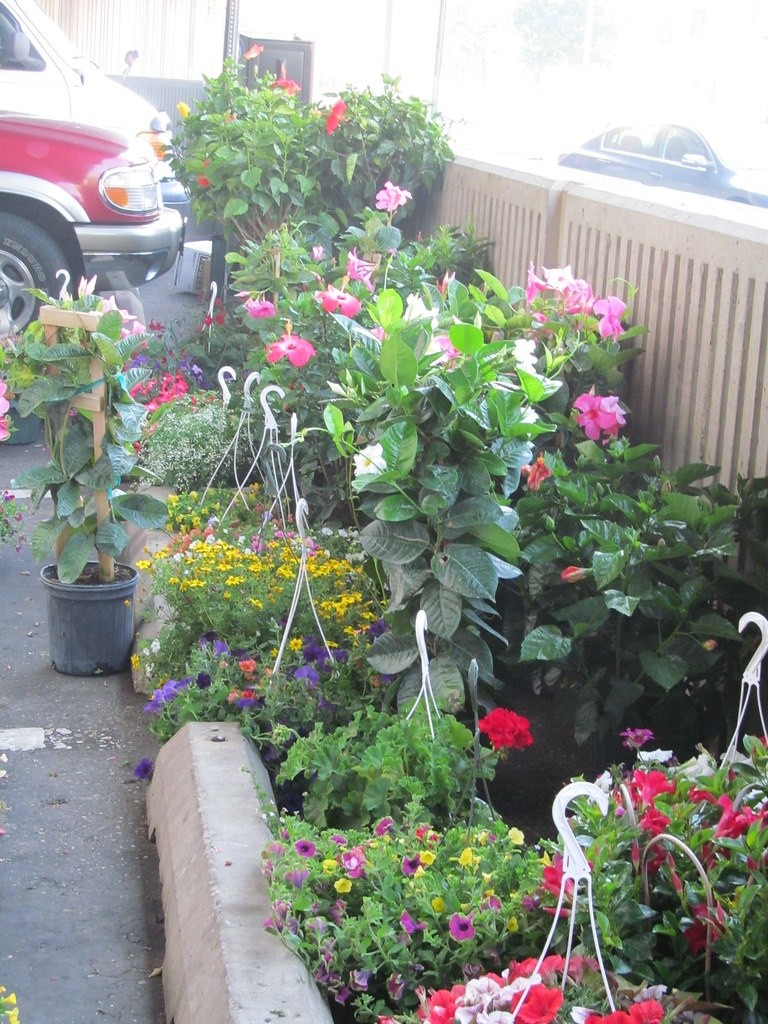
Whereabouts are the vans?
[0,0,191,339]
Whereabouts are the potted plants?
[319,289,563,795]
[0,289,175,583]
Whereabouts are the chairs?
[665,137,694,161]
[621,135,645,155]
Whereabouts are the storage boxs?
[170,241,212,294]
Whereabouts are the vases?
[39,560,138,678]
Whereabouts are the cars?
[556,117,768,211]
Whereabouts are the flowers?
[119,42,768,1024]
